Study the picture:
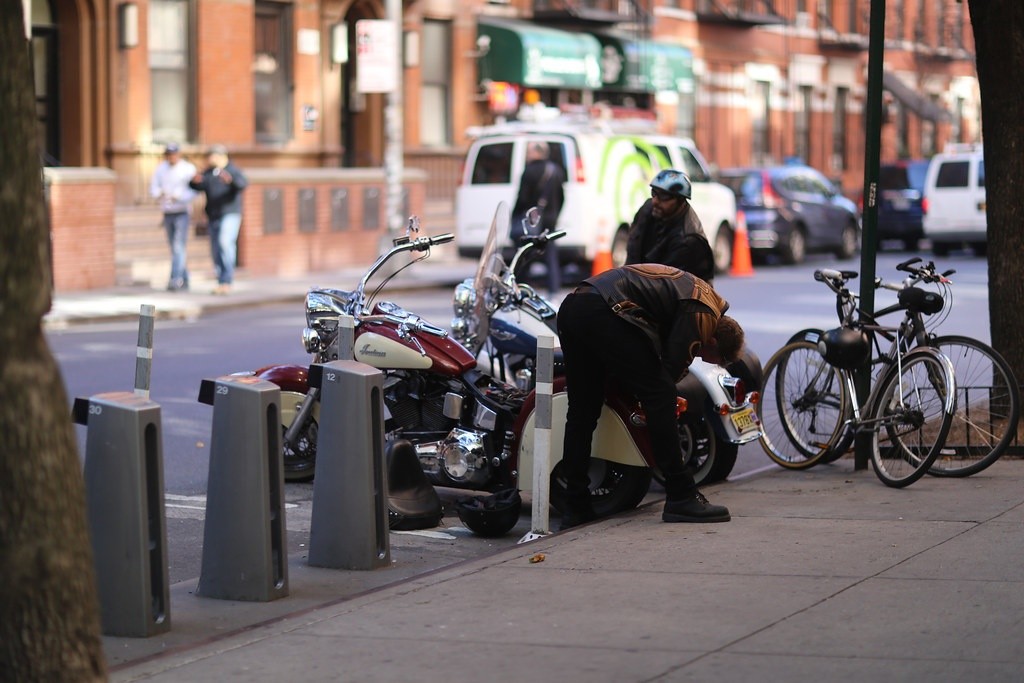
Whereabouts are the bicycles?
[752,257,1022,488]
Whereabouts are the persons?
[512,140,566,297]
[557,263,744,531]
[149,144,196,289]
[190,144,247,294]
[625,169,716,288]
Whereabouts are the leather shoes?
[662,487,731,522]
[559,505,602,530]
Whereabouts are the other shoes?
[167,284,188,295]
[213,283,233,295]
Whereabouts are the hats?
[163,143,180,158]
[205,145,227,157]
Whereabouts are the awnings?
[476,20,603,89]
[592,33,695,95]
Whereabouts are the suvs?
[711,164,862,265]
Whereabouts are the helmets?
[456,487,522,538]
[650,169,692,198]
[815,329,870,369]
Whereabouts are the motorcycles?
[228,202,766,537]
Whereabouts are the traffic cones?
[728,210,754,275]
[590,219,613,278]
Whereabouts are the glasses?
[652,188,676,202]
[718,347,734,366]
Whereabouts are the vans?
[855,151,990,256]
[452,121,736,275]
